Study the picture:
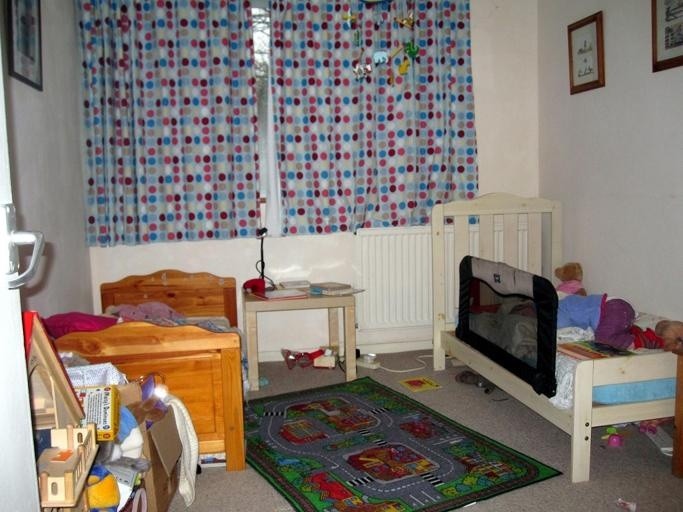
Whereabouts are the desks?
[243,292,357,391]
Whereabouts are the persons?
[557,293,663,352]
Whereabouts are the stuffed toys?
[556,264,587,296]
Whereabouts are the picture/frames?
[651,0,683,73]
[568,11,606,95]
[7,0,42,91]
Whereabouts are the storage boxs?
[143,406,183,511]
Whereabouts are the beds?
[49,269,246,470]
[431,193,681,483]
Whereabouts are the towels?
[163,394,199,507]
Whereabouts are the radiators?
[354,222,530,346]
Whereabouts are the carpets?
[242,377,563,512]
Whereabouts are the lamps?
[255,228,270,278]
